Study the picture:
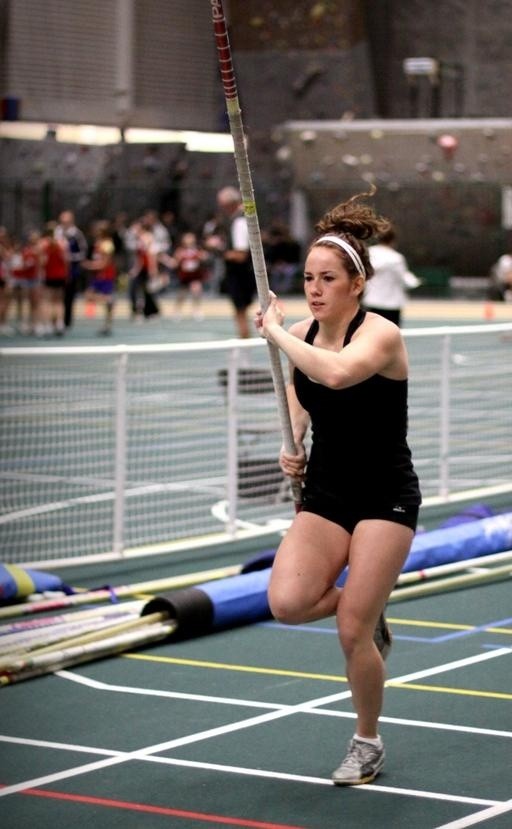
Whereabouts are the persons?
[491,253,512,303]
[0,187,258,340]
[254,200,421,786]
[363,219,421,326]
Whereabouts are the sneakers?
[376,620,392,661]
[332,733,386,784]
